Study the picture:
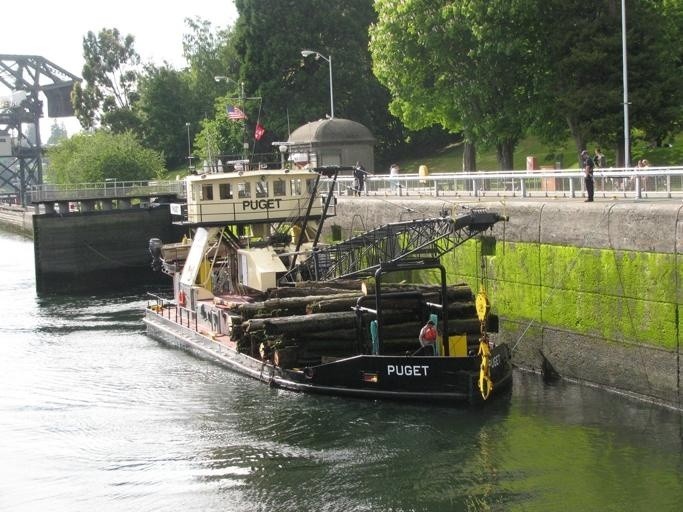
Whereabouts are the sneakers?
[585,199,592,201]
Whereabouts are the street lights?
[214,75,249,171]
[301,50,334,119]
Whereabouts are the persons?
[389,163,399,192]
[352,159,369,197]
[418,319,437,356]
[592,149,606,190]
[641,158,652,192]
[580,150,598,203]
[627,159,644,192]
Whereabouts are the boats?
[142,162,513,401]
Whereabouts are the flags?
[226,103,248,120]
[254,125,265,141]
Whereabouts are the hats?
[582,151,588,155]
